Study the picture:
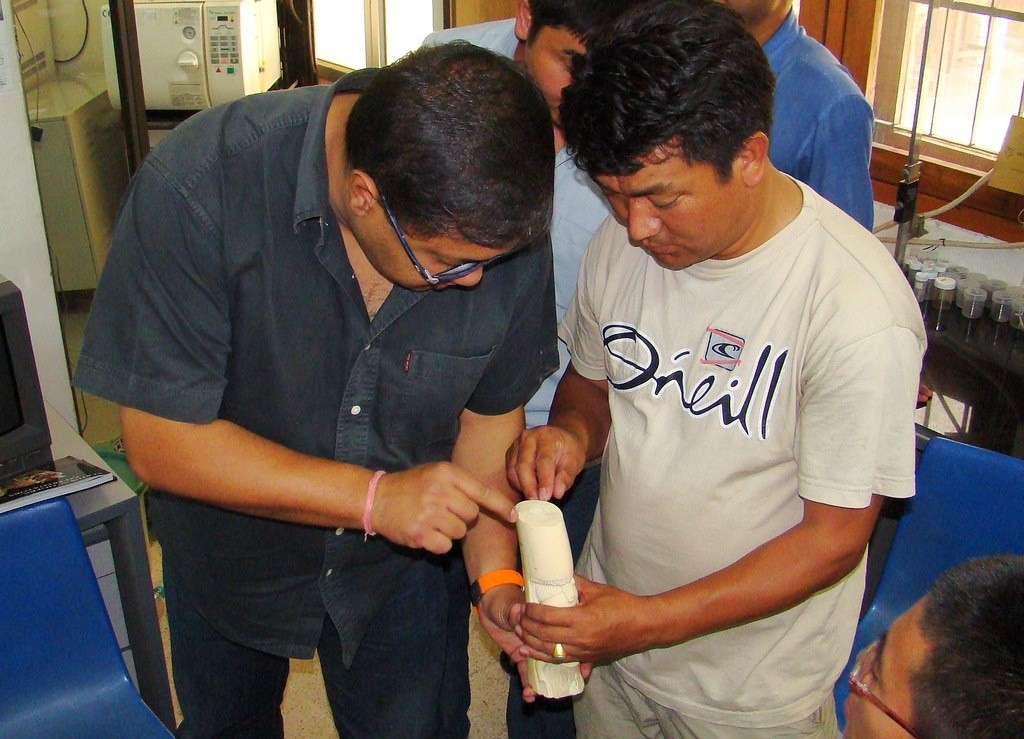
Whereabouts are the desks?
[879,305,1024,521]
[0,395,178,736]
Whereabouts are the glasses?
[377,185,534,291]
[849,640,922,739]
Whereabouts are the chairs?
[0,496,175,739]
[833,436,1024,734]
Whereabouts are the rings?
[554,644,567,661]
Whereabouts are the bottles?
[903,255,1024,329]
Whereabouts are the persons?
[423,0,928,739]
[843,556,1024,739]
[72,39,561,739]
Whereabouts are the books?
[0,456,113,514]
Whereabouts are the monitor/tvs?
[0,274,56,465]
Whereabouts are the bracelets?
[469,569,523,613]
[362,469,386,537]
